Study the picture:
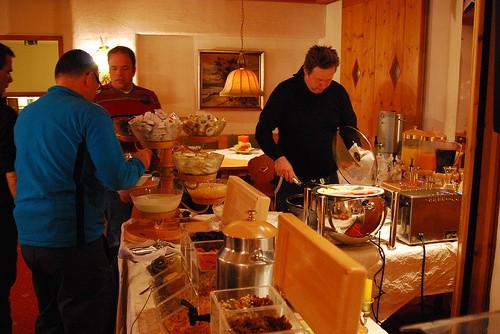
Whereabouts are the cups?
[407,165,462,197]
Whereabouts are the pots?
[311,182,387,246]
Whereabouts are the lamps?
[219,0,264,97]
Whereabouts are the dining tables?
[200,149,265,179]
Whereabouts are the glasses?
[86,69,103,95]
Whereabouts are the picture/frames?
[197,49,265,111]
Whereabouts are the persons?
[90,46,161,292]
[255,44,363,213]
[13,50,152,334]
[0,41,19,334]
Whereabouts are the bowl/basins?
[128,114,184,142]
[186,180,230,205]
[182,112,227,138]
[130,188,184,212]
[173,153,224,175]
